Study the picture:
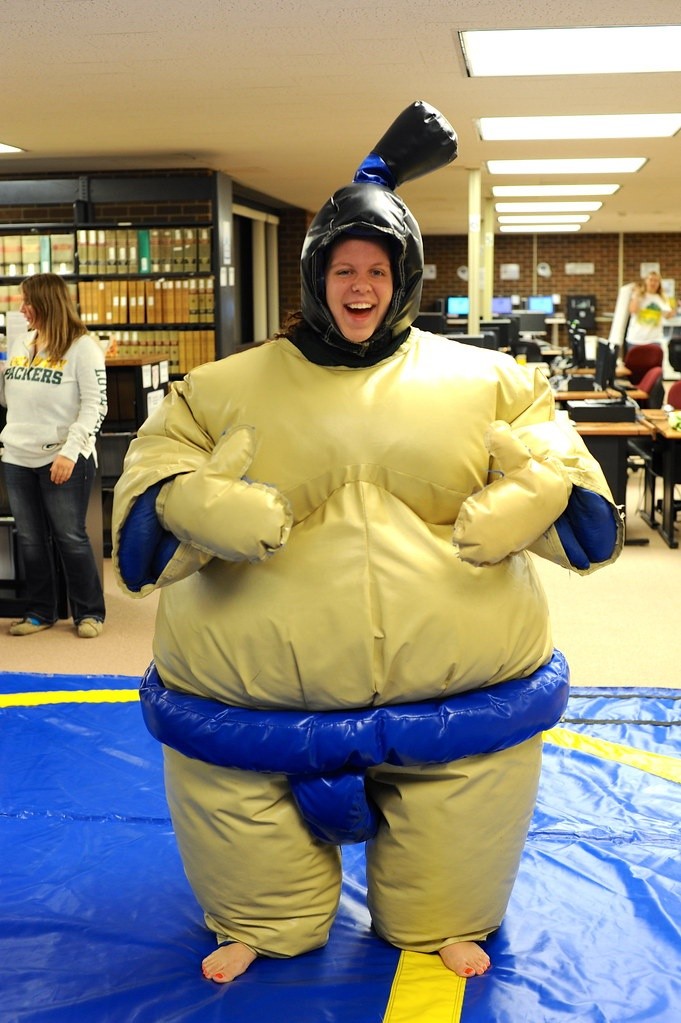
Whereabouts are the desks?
[544,317,611,346]
[555,390,650,411]
[639,409,681,531]
[556,367,633,383]
[575,422,657,547]
[650,419,681,550]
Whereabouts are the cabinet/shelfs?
[0,167,241,559]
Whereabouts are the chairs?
[657,381,681,522]
[627,366,663,473]
[625,344,664,384]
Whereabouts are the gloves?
[451,419,574,568]
[152,426,292,564]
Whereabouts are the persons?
[0,273,110,639]
[624,271,675,353]
[107,184,626,981]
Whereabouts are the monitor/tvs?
[446,295,470,317]
[492,296,512,314]
[594,340,614,391]
[527,296,553,316]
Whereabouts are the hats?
[297,98,460,359]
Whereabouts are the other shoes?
[77,617,103,638]
[9,616,54,636]
